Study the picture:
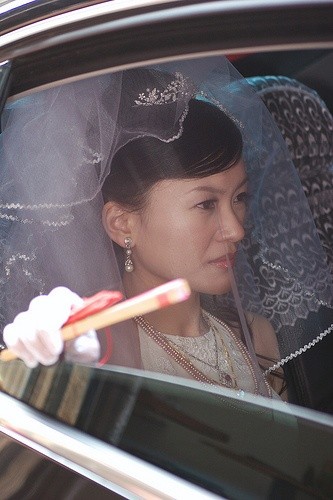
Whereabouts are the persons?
[3,66,304,434]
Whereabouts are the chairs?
[206,76,332,414]
[4,70,167,368]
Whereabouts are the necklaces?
[126,301,276,417]
